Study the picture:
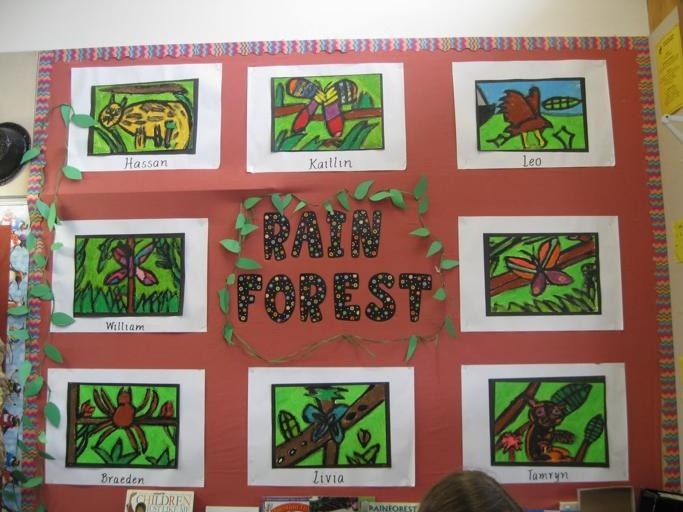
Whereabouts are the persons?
[126,493,146,512]
[416,472,524,512]
